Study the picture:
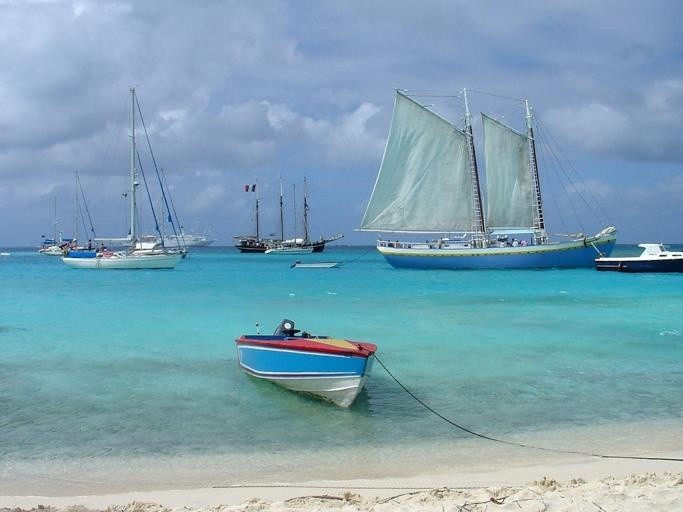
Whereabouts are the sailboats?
[62,88,187,270]
[233,173,343,252]
[93,189,157,247]
[353,88,617,268]
[38,170,100,256]
[136,170,218,249]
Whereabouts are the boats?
[594,244,683,273]
[235,319,377,408]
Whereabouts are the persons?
[87,238,105,252]
[387,237,529,250]
